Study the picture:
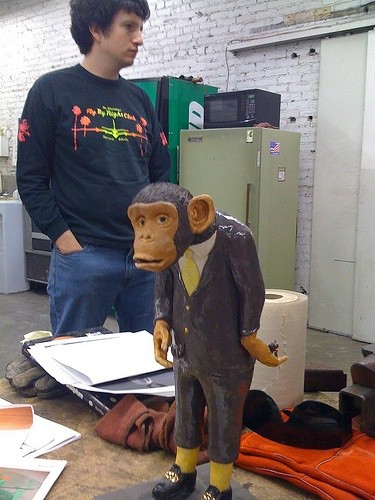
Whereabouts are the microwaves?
[203,88,281,129]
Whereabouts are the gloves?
[4,356,73,398]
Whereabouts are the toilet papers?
[249,290,311,409]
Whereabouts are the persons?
[15,0,172,337]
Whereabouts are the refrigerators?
[179,127,301,292]
